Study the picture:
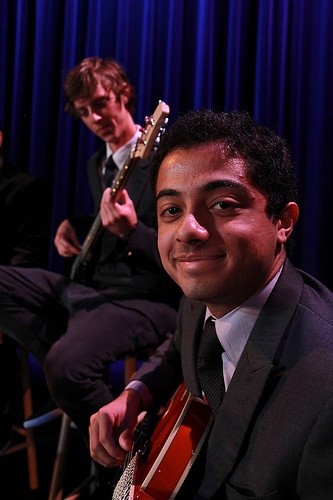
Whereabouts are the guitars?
[66,98,170,278]
[103,380,214,500]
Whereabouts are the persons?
[88,109,333,500]
[0,56,184,500]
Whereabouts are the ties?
[196,318,224,420]
[103,154,117,190]
